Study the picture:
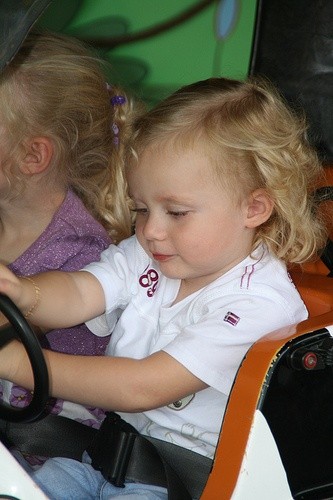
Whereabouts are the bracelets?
[310,185,333,204]
[16,274,41,320]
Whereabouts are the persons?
[0,30,333,500]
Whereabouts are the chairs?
[192,272,333,500]
